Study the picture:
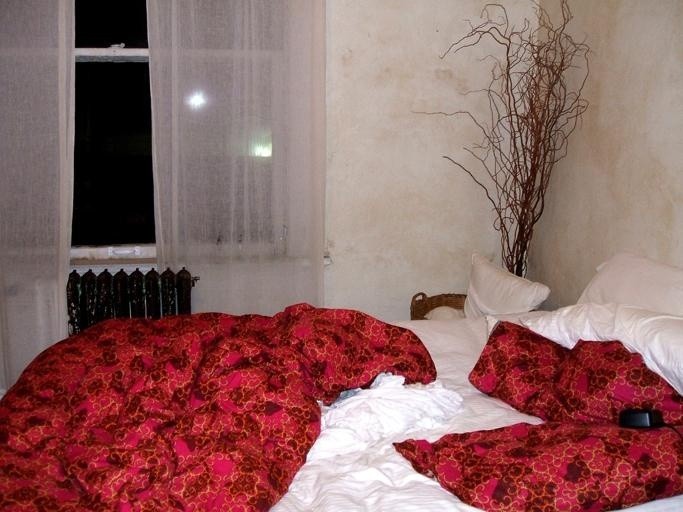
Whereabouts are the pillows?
[574,254,682,317]
[485,303,683,393]
[463,251,552,319]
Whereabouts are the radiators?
[63,266,199,336]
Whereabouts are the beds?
[0,308,683,512]
